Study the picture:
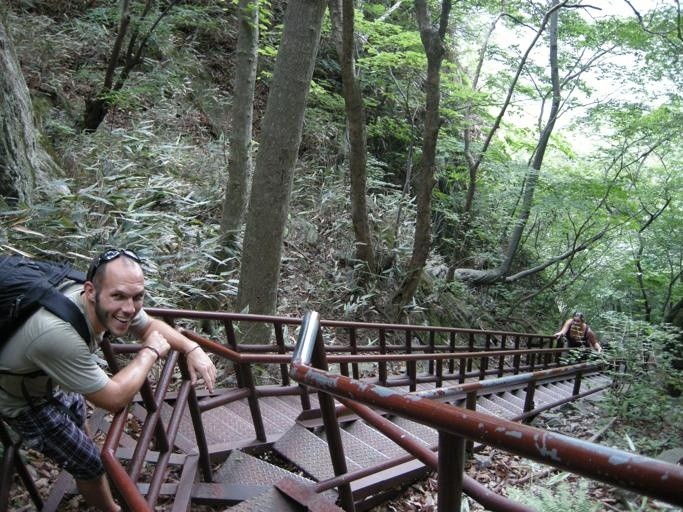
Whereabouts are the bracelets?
[142,345,160,360]
[184,344,200,357]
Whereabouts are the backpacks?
[0,253,90,351]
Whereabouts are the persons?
[553,311,603,366]
[0,247,218,511]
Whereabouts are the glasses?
[101,248,140,264]
